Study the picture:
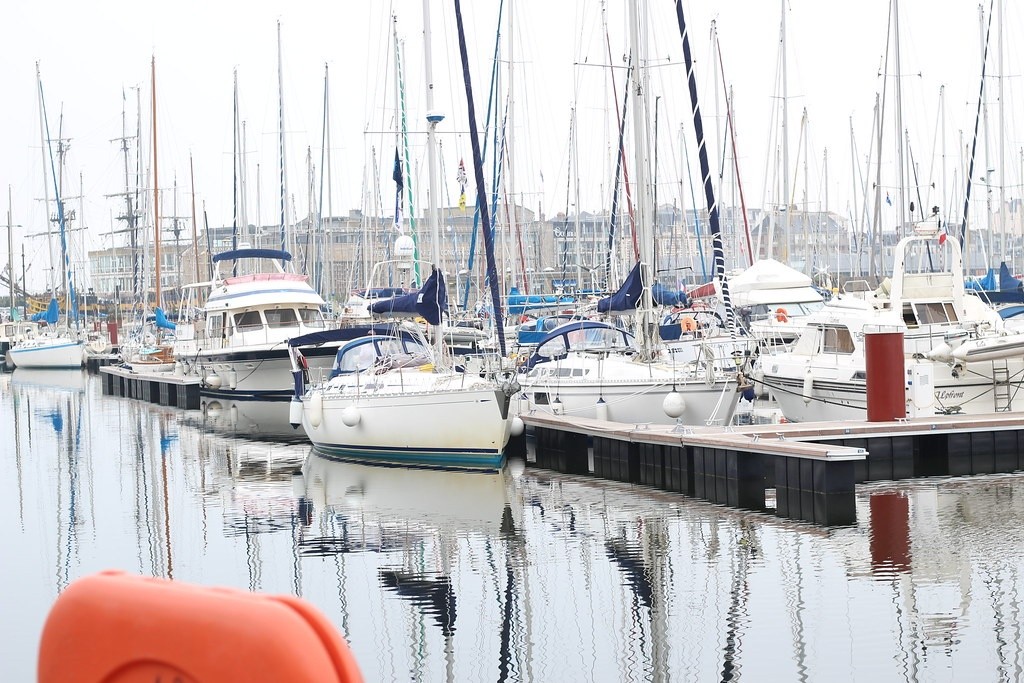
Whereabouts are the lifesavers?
[776,308,788,323]
[681,317,697,332]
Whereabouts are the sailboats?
[301,455,527,682]
[1,0,1024,472]
